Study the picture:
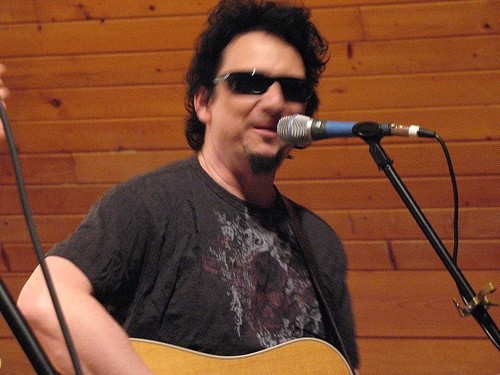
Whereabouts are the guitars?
[128,337,356,375]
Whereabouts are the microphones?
[277,115,435,146]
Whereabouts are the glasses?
[213,71,314,102]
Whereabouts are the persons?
[0,59,11,146]
[17,0,361,375]
